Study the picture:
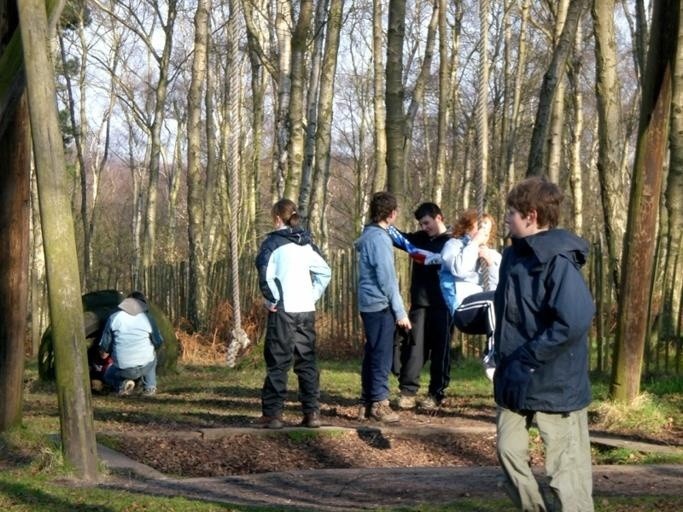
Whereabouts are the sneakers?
[396,394,417,410]
[118,379,134,397]
[249,415,283,429]
[359,398,400,423]
[419,394,442,411]
[297,411,321,428]
[142,388,157,396]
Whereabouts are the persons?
[351,192,413,423]
[438,209,503,382]
[252,198,331,430]
[86,289,164,400]
[385,202,456,408]
[494,177,597,512]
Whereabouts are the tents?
[37,288,184,383]
